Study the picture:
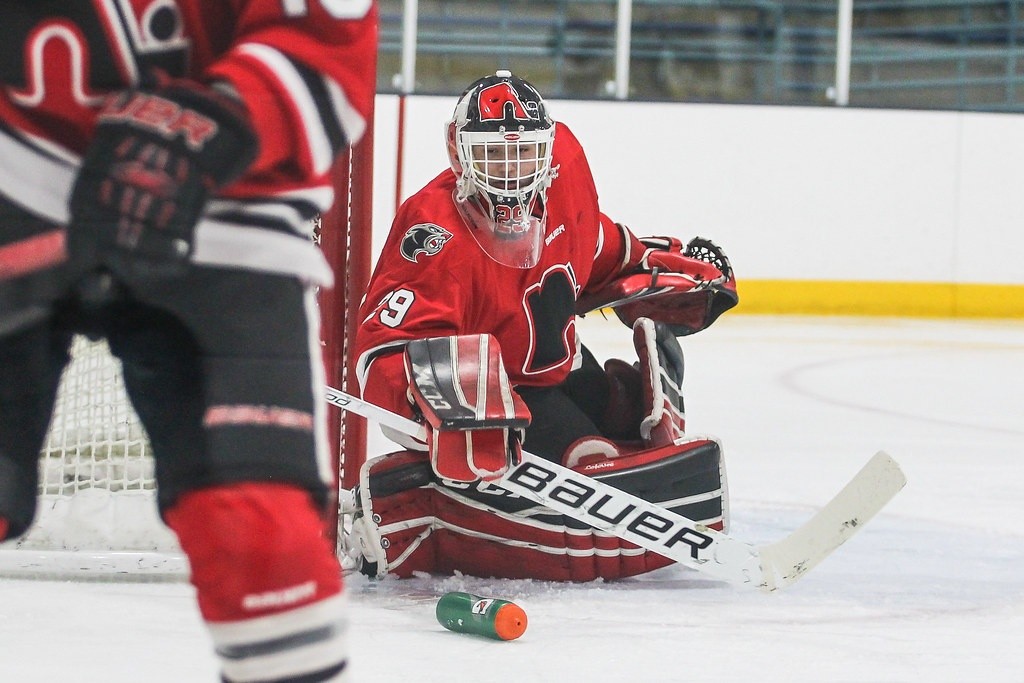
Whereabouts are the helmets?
[453,69,553,218]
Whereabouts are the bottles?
[436,592,527,641]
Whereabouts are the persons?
[1,1,380,683]
[342,70,739,584]
[549,0,817,103]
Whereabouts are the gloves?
[51,70,264,341]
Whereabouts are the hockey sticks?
[0,228,68,281]
[323,383,908,595]
